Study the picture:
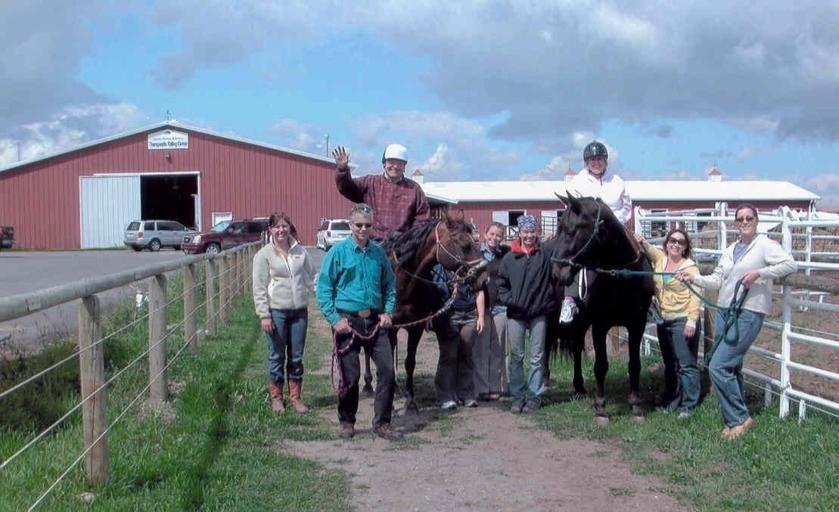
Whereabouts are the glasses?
[668,237,686,245]
[736,216,754,221]
[353,221,372,227]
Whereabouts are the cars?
[0,226,15,248]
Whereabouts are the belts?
[346,310,380,317]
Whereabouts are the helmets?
[382,144,409,164]
[583,142,608,160]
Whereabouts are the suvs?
[181,217,302,260]
[122,219,203,252]
[315,218,352,251]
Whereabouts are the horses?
[362,208,493,417]
[547,189,655,426]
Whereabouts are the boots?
[286,378,308,413]
[268,379,285,414]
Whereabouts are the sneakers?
[655,403,753,441]
[435,393,540,413]
[337,423,354,438]
[371,423,402,440]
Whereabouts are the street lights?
[325,134,330,157]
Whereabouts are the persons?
[315,202,402,440]
[331,144,431,243]
[635,230,702,419]
[473,222,510,401]
[252,211,318,414]
[675,204,798,439]
[495,215,558,414]
[425,264,485,409]
[558,141,663,326]
